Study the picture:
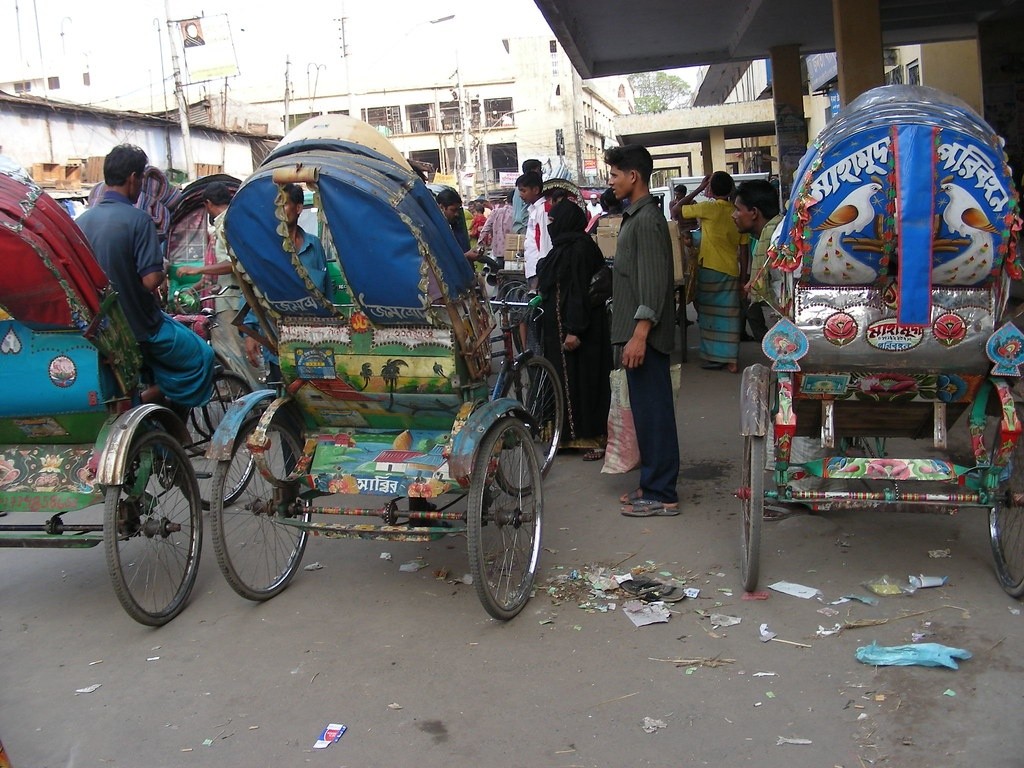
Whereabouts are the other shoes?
[742,334,754,340]
[700,360,722,367]
[754,336,763,342]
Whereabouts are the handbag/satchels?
[601,368,642,474]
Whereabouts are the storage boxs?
[503,234,527,270]
[589,216,684,283]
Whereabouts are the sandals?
[619,486,643,504]
[620,496,680,517]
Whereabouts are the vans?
[648,170,769,229]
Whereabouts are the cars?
[578,189,603,235]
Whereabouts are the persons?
[70,143,787,518]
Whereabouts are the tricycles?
[1,169,263,626]
[204,114,569,620]
[734,82,1023,599]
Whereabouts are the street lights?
[448,108,526,206]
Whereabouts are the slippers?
[583,448,606,461]
[763,505,792,520]
[763,496,777,506]
